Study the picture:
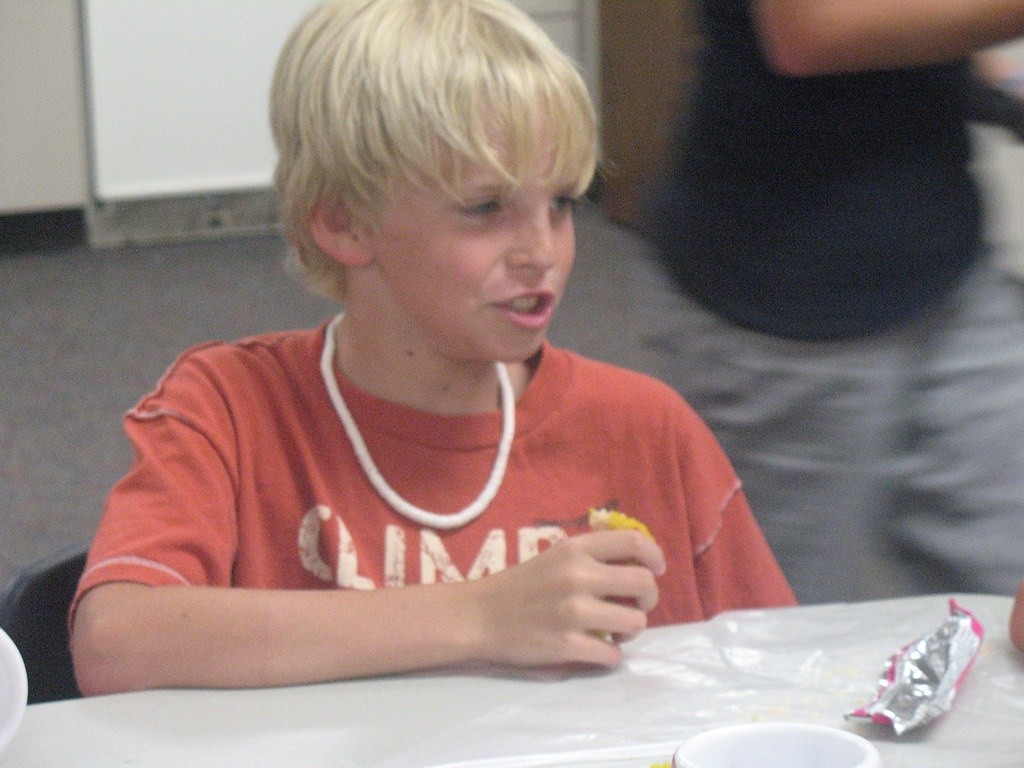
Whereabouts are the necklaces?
[318,309,519,532]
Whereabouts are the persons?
[62,0,800,700]
[644,0,1023,654]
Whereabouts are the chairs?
[0,541,90,705]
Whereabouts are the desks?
[0,594,1023,768]
